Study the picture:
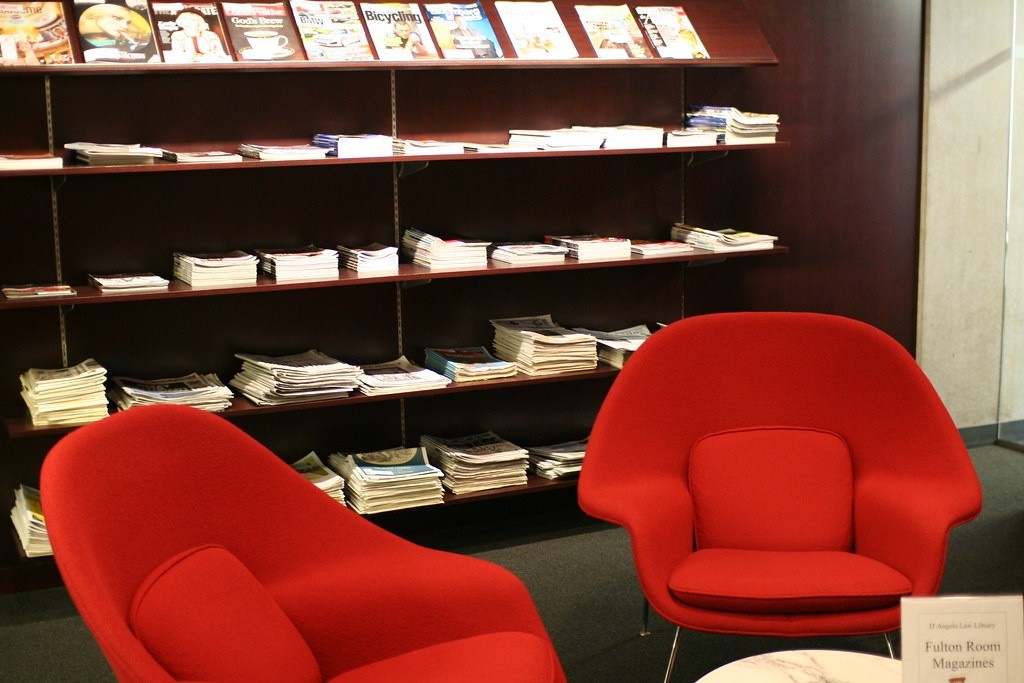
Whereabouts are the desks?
[695,649,905,683]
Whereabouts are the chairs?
[38,406,569,682]
[577,310,983,683]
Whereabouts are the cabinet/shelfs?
[0,0,787,589]
